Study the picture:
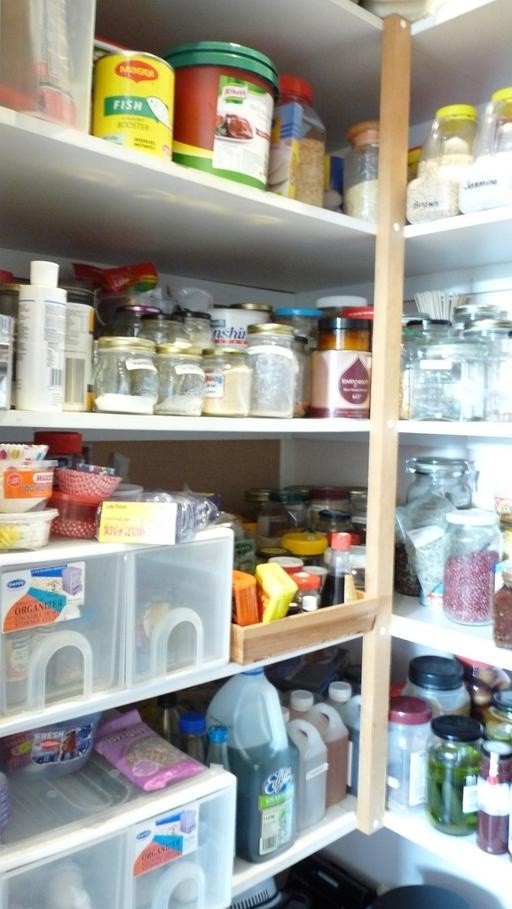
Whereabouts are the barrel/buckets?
[203,666,300,864]
[323,681,362,799]
[280,704,328,831]
[158,41,282,191]
[1,1,98,135]
[289,689,351,808]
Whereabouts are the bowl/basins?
[0,442,144,552]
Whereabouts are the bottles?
[341,116,378,226]
[399,303,512,424]
[283,68,331,211]
[441,509,511,650]
[148,691,229,778]
[400,454,476,517]
[224,484,369,618]
[2,257,375,420]
[384,653,512,863]
[34,431,86,487]
[406,87,510,225]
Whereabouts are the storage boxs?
[0,523,237,908]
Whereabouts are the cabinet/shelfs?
[0,0,511,909]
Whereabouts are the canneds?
[91,50,176,163]
[427,689,512,855]
[92,294,375,419]
[253,546,321,616]
